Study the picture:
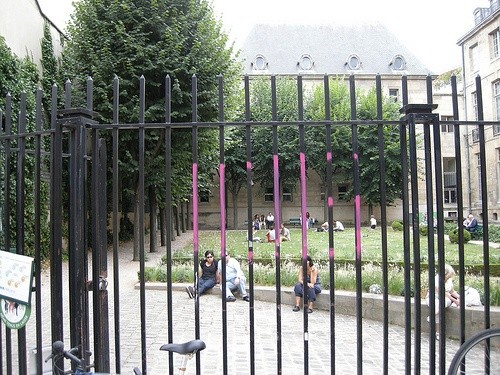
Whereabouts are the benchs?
[445,217,465,222]
[470,224,483,240]
[244,220,269,229]
[289,219,318,228]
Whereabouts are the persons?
[370,215,377,229]
[186,250,218,298]
[266,226,275,243]
[426,263,459,339]
[281,224,290,242]
[464,214,477,231]
[253,214,266,230]
[266,212,275,228]
[247,225,263,243]
[293,255,322,313]
[333,220,344,231]
[320,221,329,232]
[216,249,250,301]
[306,212,312,229]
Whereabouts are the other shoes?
[186,286,194,299]
[436,332,439,340]
[308,308,313,313]
[293,306,300,311]
[243,296,250,302]
[226,296,236,302]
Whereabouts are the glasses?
[207,256,212,258]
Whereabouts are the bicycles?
[447,327,500,375]
[42,340,207,375]
